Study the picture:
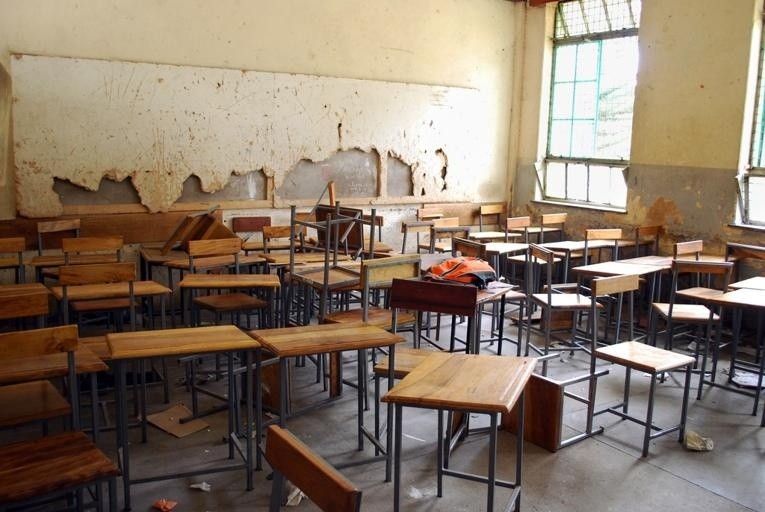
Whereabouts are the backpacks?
[422,256,497,290]
